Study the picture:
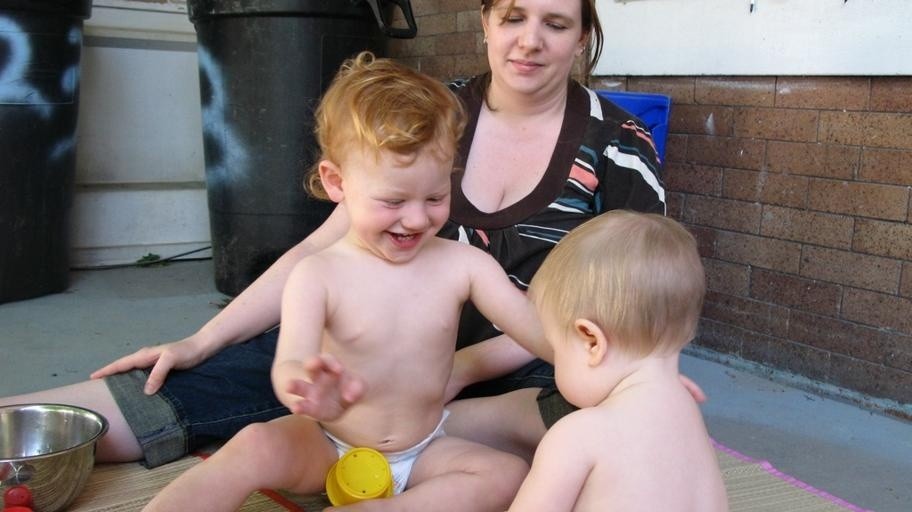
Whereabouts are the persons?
[0,0,667,470]
[138,49,707,512]
[504,208,729,512]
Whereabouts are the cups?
[325,448,393,507]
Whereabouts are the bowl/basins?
[0,403,109,512]
